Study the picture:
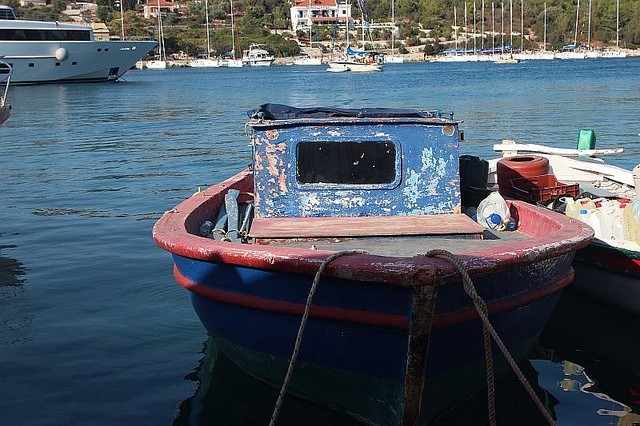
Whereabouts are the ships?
[0,5,158,86]
[242,45,275,67]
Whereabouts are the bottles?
[506,217,517,230]
[481,200,504,230]
[466,206,479,222]
[557,196,640,247]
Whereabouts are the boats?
[329,61,384,72]
[494,59,520,64]
[153,110,596,425]
[485,153,640,374]
